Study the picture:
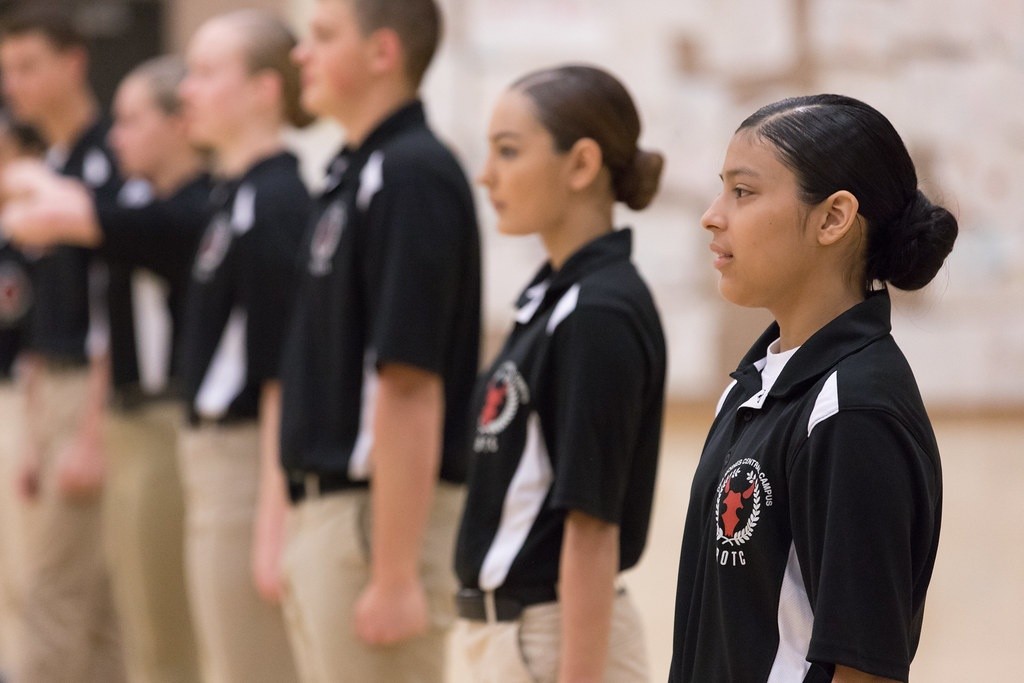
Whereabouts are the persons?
[444,63,668,683]
[0,0,483,683]
[666,94,959,683]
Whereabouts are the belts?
[187,413,257,425]
[287,472,465,503]
[454,591,556,620]
